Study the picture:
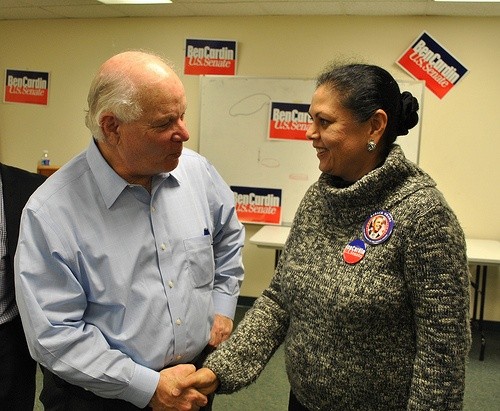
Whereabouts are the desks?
[250,225,500,361]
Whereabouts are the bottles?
[40,151,50,166]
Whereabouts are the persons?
[368,216,384,239]
[171,63,471,411]
[0,162,47,411]
[13,51,246,411]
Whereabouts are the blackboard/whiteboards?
[200,76,421,230]
